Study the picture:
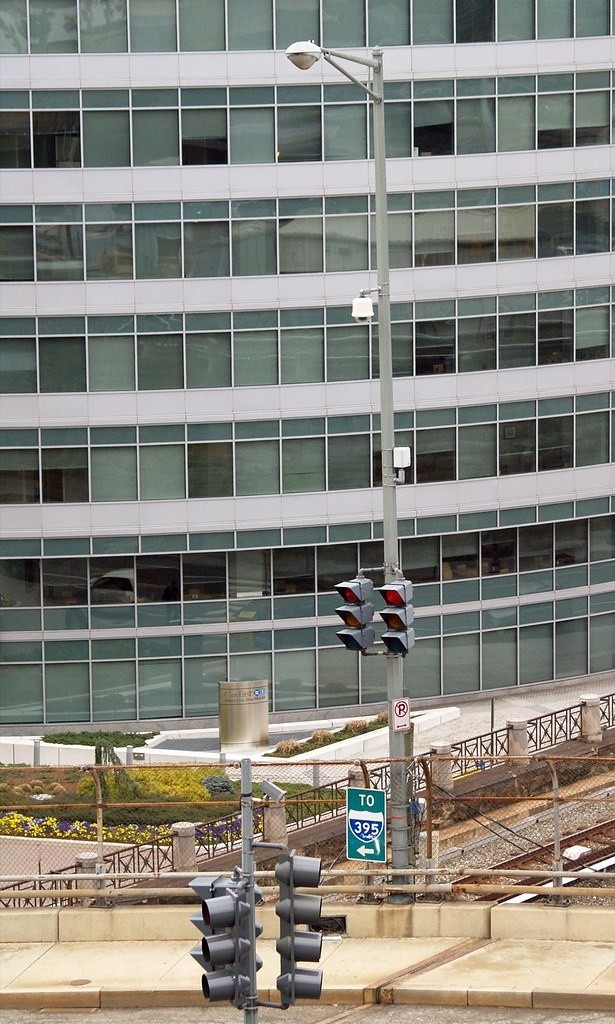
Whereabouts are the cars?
[90,569,138,606]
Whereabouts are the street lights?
[285,39,428,894]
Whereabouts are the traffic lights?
[271,854,328,1005]
[201,889,251,1009]
[328,569,377,656]
[186,874,263,983]
[369,574,419,656]
